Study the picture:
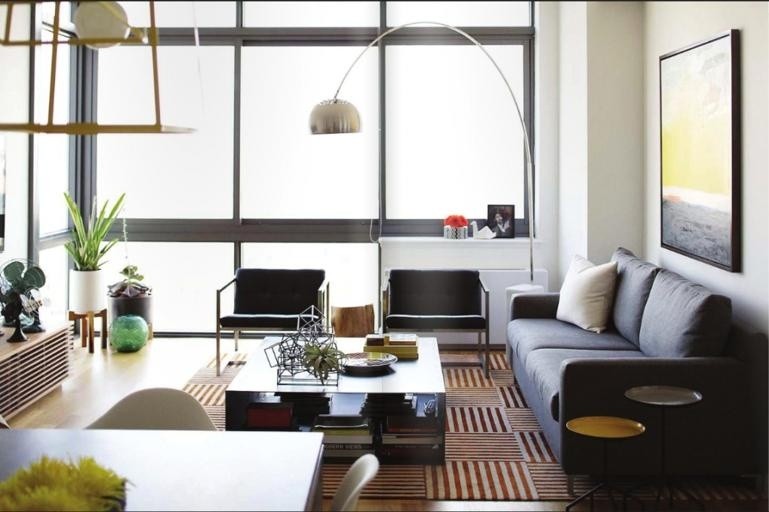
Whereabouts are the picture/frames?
[659,28,742,274]
[487,204,515,239]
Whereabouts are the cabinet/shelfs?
[0,321,74,426]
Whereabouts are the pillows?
[557,252,619,335]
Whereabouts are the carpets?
[179,349,768,500]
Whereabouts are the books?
[363,333,419,361]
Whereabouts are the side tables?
[330,299,375,337]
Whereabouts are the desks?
[0,428,325,512]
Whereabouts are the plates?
[335,351,398,370]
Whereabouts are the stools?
[566,418,648,512]
[625,387,706,512]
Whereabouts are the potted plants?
[66,191,125,315]
[108,266,153,325]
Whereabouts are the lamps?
[308,22,542,362]
[74,1,129,50]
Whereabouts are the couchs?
[507,247,769,494]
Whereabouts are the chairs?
[328,453,380,512]
[85,388,218,431]
[385,268,490,378]
[216,269,329,377]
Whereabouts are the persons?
[493,213,510,232]
